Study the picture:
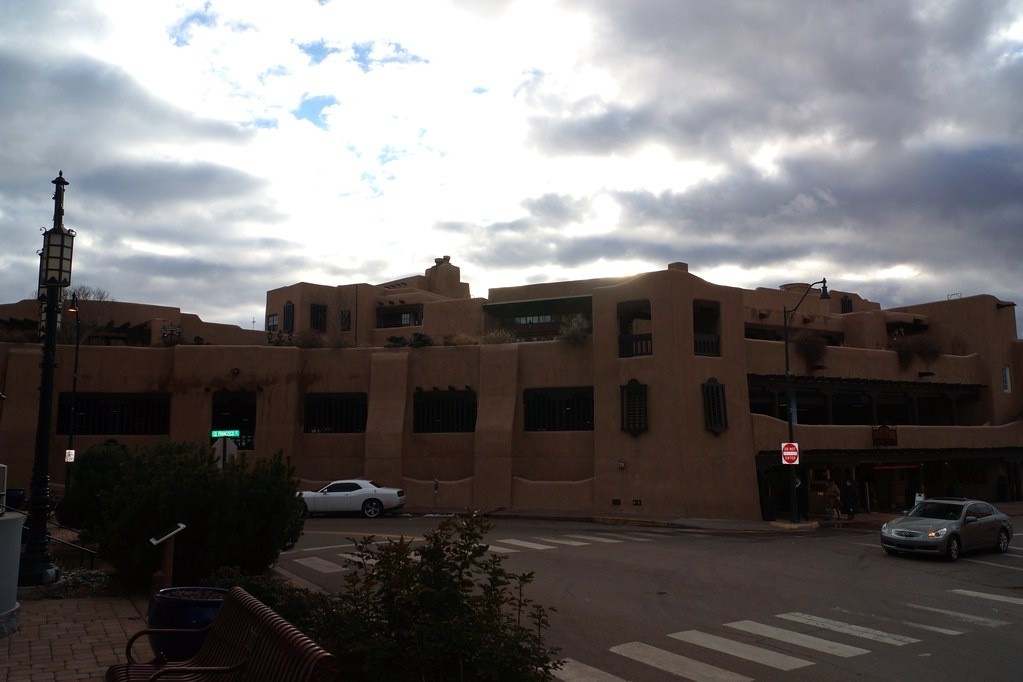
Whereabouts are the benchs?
[105,585,334,682]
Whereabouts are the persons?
[796,478,809,520]
[845,480,856,521]
[823,480,841,520]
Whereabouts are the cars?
[880,497,1013,561]
[296,479,405,520]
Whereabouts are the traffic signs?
[211,430,240,437]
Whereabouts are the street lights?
[6,170,77,585]
[783,277,831,522]
[61,293,80,499]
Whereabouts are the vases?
[146,586,228,663]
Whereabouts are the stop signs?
[781,442,799,464]
